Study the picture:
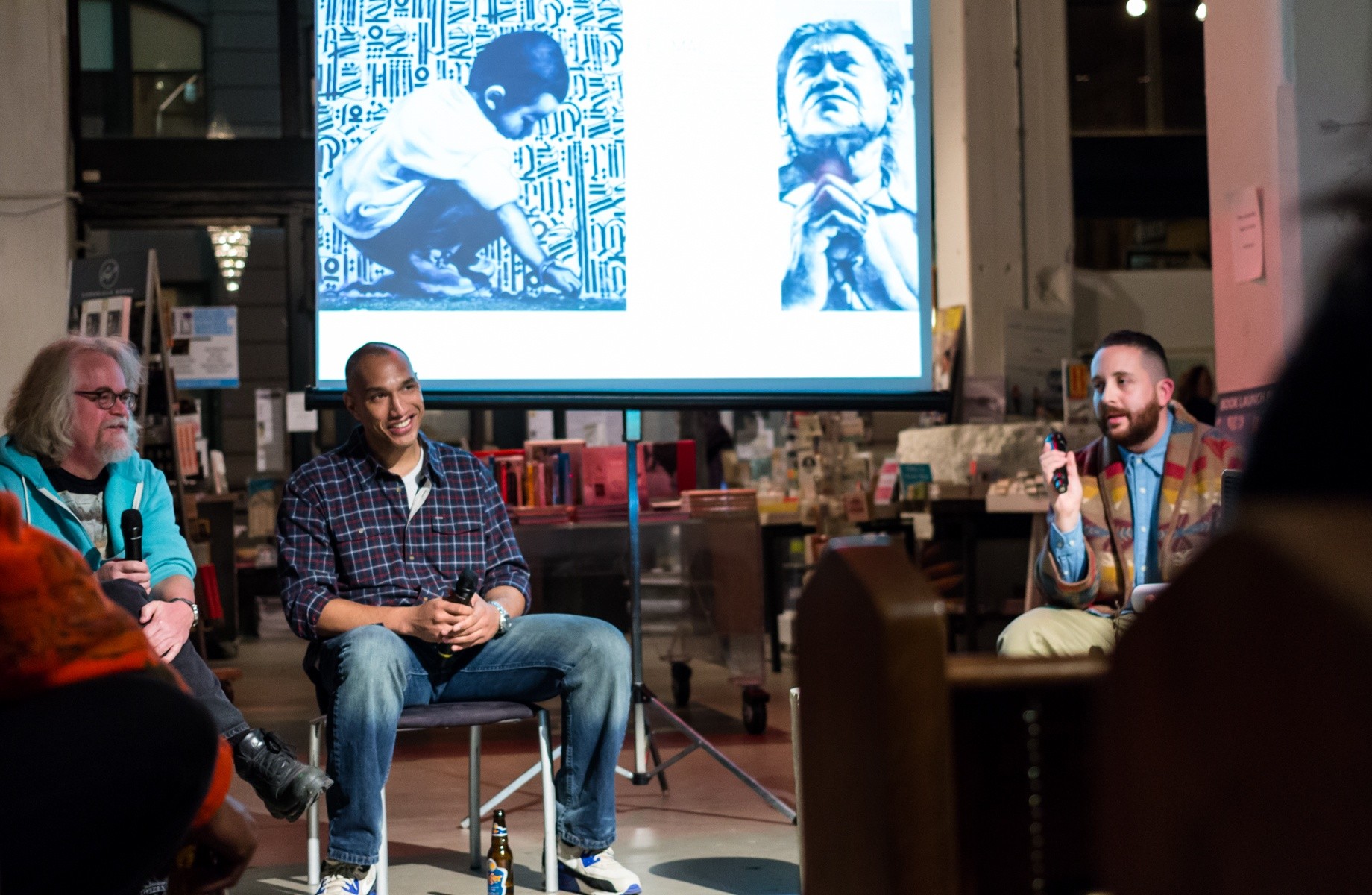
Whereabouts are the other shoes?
[395,250,475,296]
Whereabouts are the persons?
[1175,363,1217,426]
[319,30,581,298]
[997,328,1248,660]
[0,337,334,895]
[772,18,917,310]
[281,341,639,895]
[1088,183,1372,895]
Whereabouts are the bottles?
[486,809,514,895]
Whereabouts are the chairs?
[308,659,559,895]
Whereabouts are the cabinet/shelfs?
[1019,0,1214,354]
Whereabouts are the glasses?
[75,389,138,411]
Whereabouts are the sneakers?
[541,836,642,895]
[234,727,335,822]
[315,859,376,895]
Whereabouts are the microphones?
[437,567,480,658]
[121,509,144,574]
[1044,431,1069,493]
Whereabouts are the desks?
[185,491,239,639]
[511,489,1106,895]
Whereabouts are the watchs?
[487,601,511,632]
[168,597,198,627]
